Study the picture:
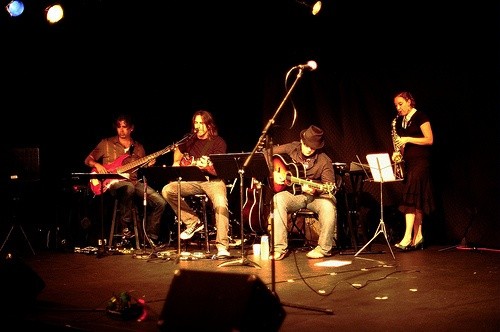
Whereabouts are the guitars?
[267,153,339,196]
[179,153,217,176]
[241,137,267,234]
[88,144,178,196]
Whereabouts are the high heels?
[395,236,425,252]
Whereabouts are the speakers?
[158,269,286,332]
[0,258,46,300]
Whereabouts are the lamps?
[299,0,322,15]
[4,0,26,17]
[43,4,64,24]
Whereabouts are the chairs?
[270,171,344,250]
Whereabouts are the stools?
[108,193,140,250]
[187,195,215,253]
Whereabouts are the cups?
[253,244,261,256]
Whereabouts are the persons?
[161,110,231,259]
[84,115,167,249]
[391,90,434,253]
[258,125,338,261]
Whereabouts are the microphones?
[296,61,318,71]
[189,129,198,140]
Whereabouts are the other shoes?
[306,247,327,258]
[121,228,131,238]
[269,248,289,260]
[149,238,169,251]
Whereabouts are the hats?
[300,125,326,149]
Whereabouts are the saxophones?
[391,111,405,163]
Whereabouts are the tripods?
[209,152,272,268]
[352,153,405,260]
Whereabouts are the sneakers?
[217,243,231,257]
[180,218,204,239]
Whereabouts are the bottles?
[260,235,269,260]
